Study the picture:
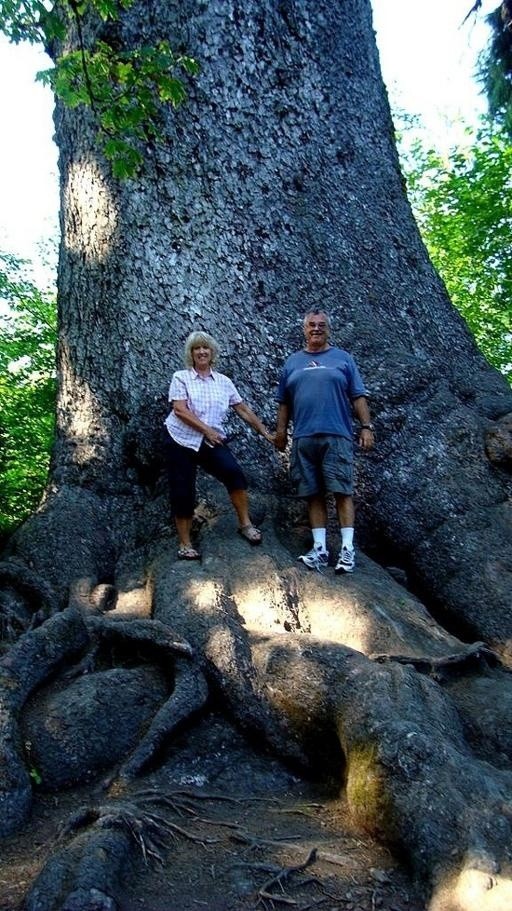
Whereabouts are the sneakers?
[296,541,330,571]
[333,545,357,575]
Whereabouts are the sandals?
[176,545,202,562]
[236,524,264,546]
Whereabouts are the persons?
[162,331,275,560]
[276,308,376,573]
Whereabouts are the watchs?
[361,423,375,431]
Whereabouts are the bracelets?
[264,430,269,437]
